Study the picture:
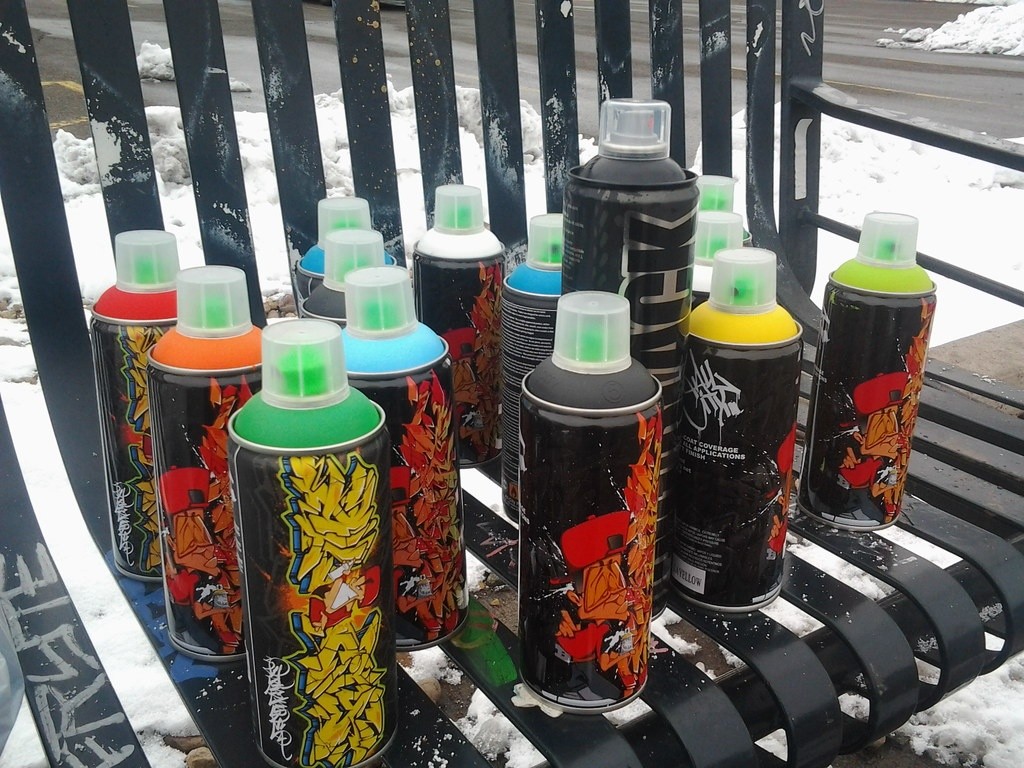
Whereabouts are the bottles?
[339,265,472,652]
[671,247,802,612]
[697,174,753,250]
[691,211,745,307]
[518,291,663,713]
[298,226,384,330]
[297,197,396,304]
[413,183,505,469]
[798,210,937,530]
[562,101,699,587]
[228,319,399,768]
[501,214,561,521]
[146,266,263,661]
[89,232,183,581]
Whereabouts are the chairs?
[0,0,1024,768]
